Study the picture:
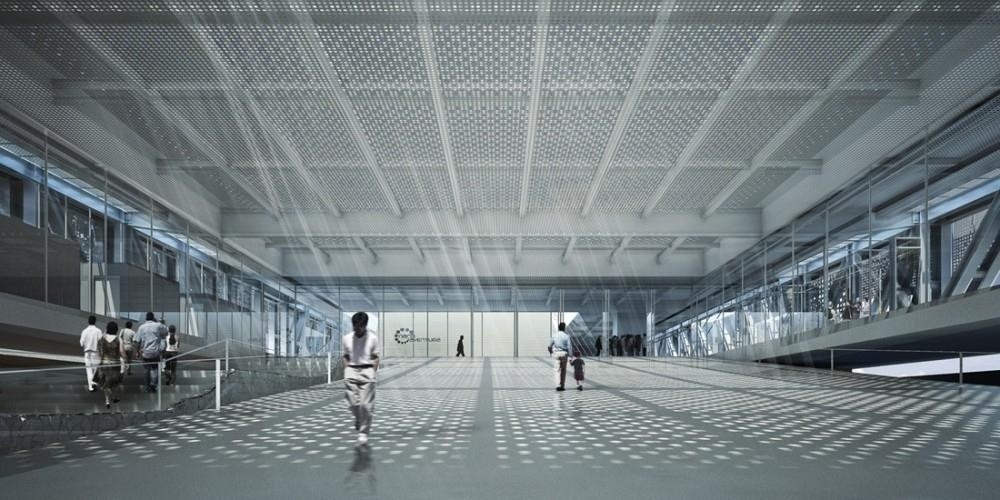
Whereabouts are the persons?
[569,351,586,391]
[80,311,180,409]
[342,311,381,446]
[547,322,573,391]
[594,333,647,357]
[456,335,465,357]
